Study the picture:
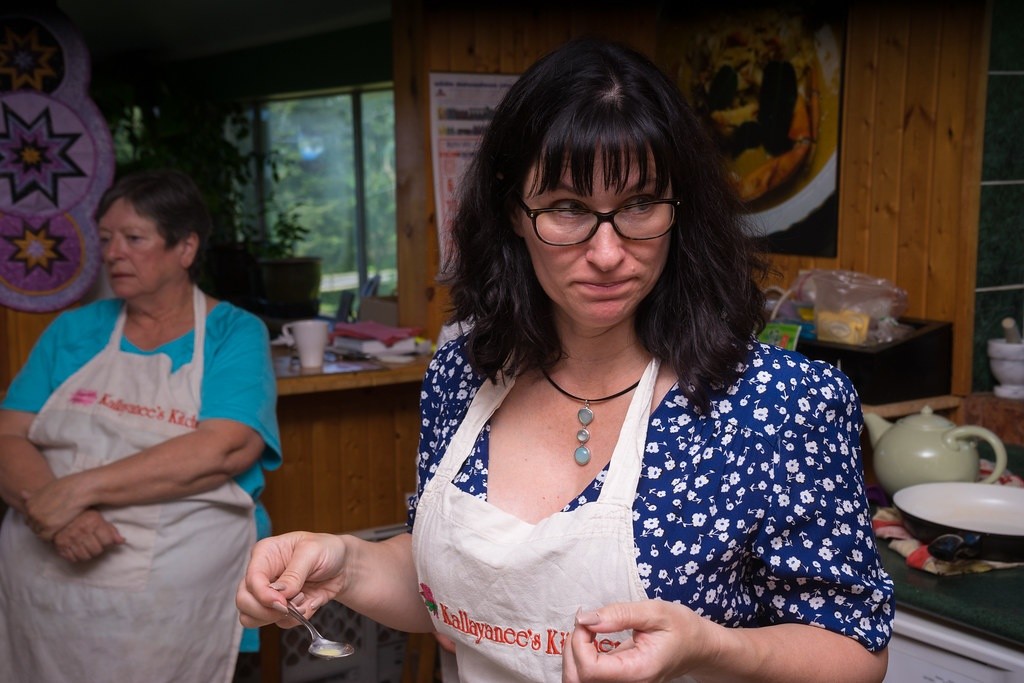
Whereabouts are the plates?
[377,355,416,367]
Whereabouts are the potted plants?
[261,208,322,300]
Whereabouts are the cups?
[282,321,328,369]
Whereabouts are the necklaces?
[542,368,640,466]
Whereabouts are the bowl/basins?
[732,20,841,238]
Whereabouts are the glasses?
[508,182,681,246]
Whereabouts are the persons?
[0,167,284,683]
[237,36,896,683]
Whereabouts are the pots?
[891,481,1024,563]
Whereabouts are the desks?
[260,333,438,683]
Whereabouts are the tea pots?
[862,405,1008,500]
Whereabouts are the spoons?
[286,599,356,662]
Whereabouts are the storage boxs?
[789,313,953,405]
